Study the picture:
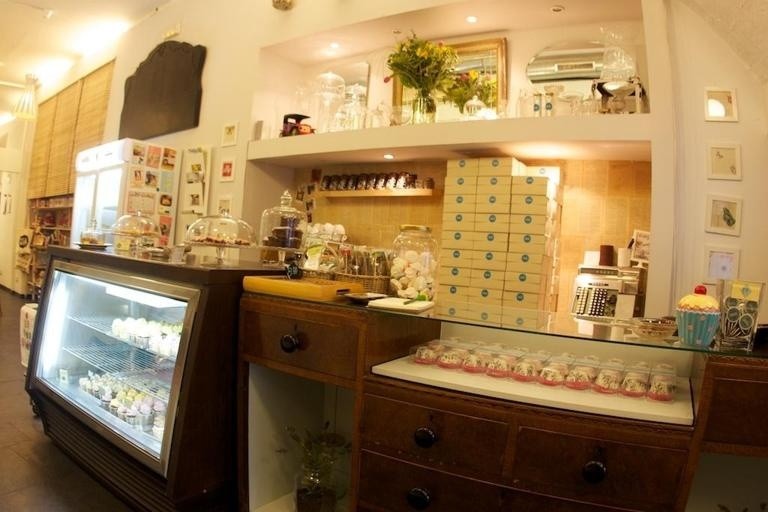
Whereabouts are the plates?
[344,292,387,304]
[72,242,114,249]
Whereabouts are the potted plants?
[274,419,352,511]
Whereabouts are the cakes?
[675,281,722,349]
[414,331,677,402]
[76,313,184,439]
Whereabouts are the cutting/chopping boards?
[368,297,436,314]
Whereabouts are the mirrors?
[315,59,373,135]
[389,33,512,125]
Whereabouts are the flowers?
[383,31,457,97]
[442,68,500,119]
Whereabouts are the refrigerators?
[67,136,184,256]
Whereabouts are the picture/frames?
[706,143,746,183]
[700,242,744,287]
[700,85,740,126]
[214,121,240,223]
[701,192,748,239]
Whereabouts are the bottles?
[311,72,346,134]
[388,224,441,302]
[531,93,543,118]
[259,190,308,262]
[463,95,488,121]
[543,91,554,118]
[337,83,374,130]
[80,219,105,247]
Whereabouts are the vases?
[410,91,440,125]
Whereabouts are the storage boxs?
[433,152,566,329]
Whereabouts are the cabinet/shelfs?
[23,190,74,304]
[24,252,283,512]
[357,302,717,512]
[233,282,444,511]
[685,346,768,460]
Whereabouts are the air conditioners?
[523,49,610,86]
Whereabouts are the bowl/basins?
[629,316,677,342]
[386,105,413,125]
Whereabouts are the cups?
[675,306,720,348]
[617,248,630,267]
[599,244,613,266]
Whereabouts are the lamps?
[13,71,43,124]
